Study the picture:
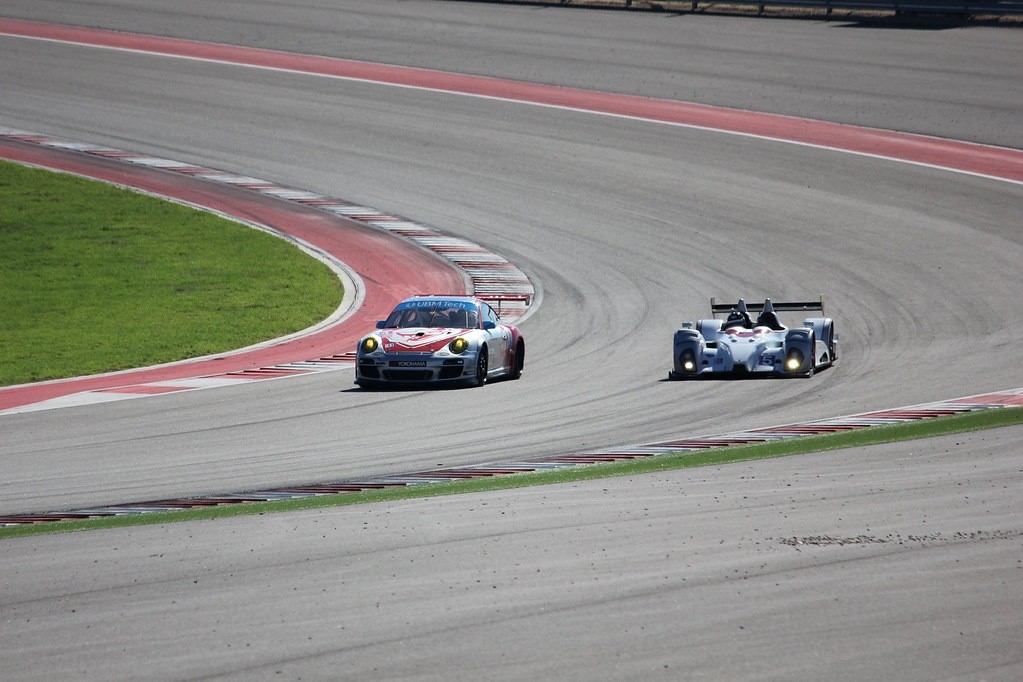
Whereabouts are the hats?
[763,298,774,313]
[737,299,748,312]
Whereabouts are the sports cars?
[354,294,525,387]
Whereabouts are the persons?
[726,312,746,328]
